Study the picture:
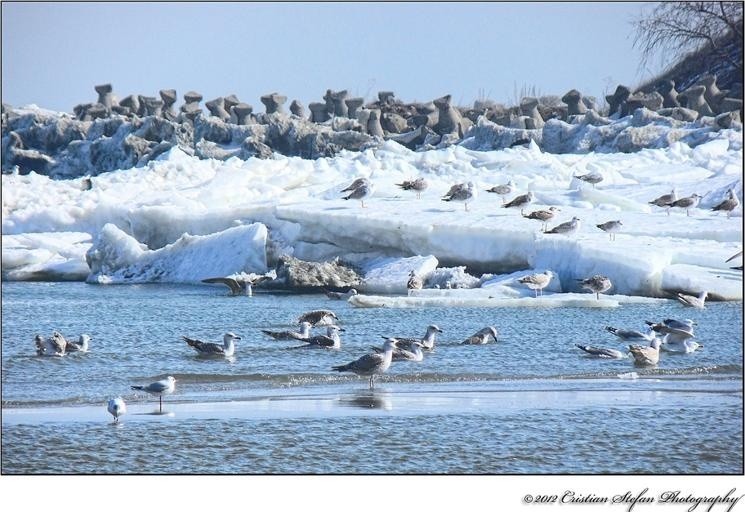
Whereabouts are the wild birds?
[259,308,443,390]
[34,331,91,358]
[518,270,553,298]
[573,317,704,366]
[461,325,499,347]
[646,187,740,219]
[675,290,709,309]
[394,176,428,200]
[442,171,622,240]
[338,175,375,208]
[407,269,422,290]
[182,332,241,357]
[108,374,177,423]
[201,276,270,296]
[573,274,613,299]
[320,285,358,301]
